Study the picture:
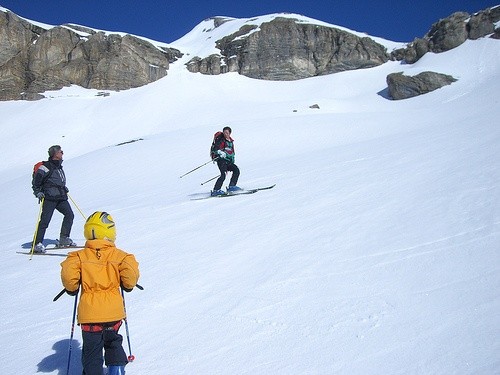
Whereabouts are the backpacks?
[211,132,226,161]
[32,161,54,194]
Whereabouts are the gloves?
[37,192,45,199]
[218,150,226,159]
[65,186,69,192]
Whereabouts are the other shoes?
[212,189,224,195]
[106,366,125,375]
[228,185,241,191]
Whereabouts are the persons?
[33,145,77,255]
[61,211,140,375]
[211,126,241,196]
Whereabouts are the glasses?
[61,151,63,154]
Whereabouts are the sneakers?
[59,236,73,245]
[32,243,46,254]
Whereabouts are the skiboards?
[15,246,85,256]
[189,184,276,200]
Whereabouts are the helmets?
[84,211,116,243]
